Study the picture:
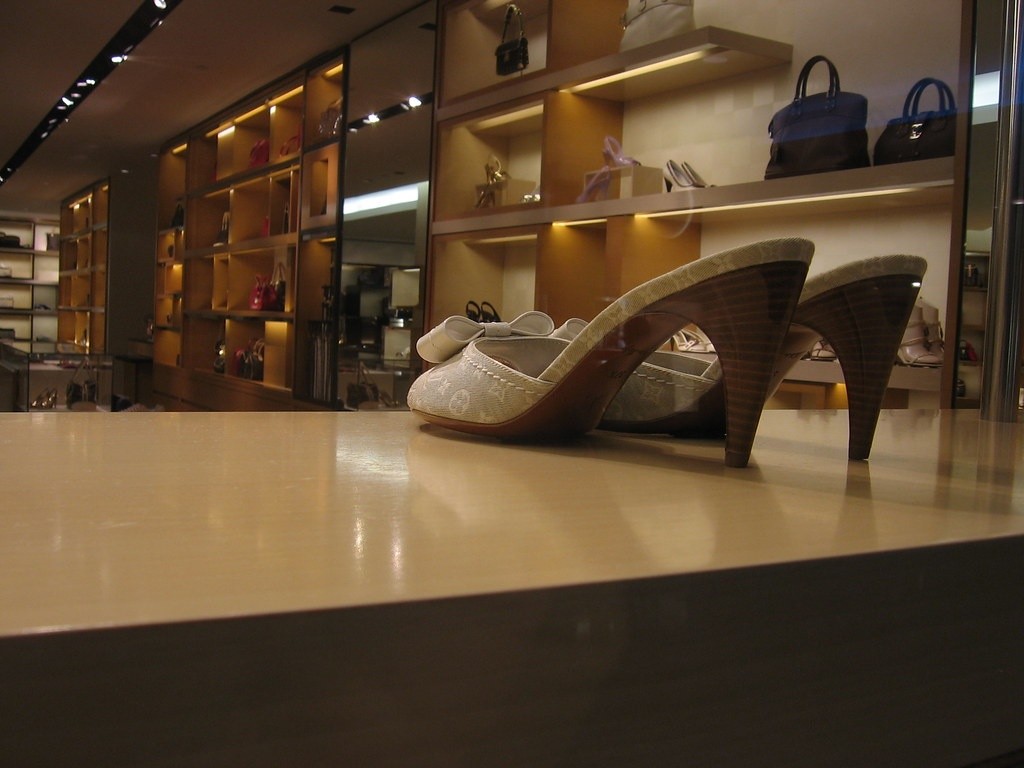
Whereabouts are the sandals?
[802,337,837,362]
[896,304,944,368]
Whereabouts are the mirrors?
[331,0,448,411]
[937,0,1024,413]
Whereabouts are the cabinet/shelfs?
[0,219,61,340]
[58,177,154,353]
[422,0,954,408]
[151,42,351,412]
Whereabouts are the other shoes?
[670,327,715,354]
[463,300,499,324]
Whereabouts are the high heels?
[408,238,815,469]
[470,155,511,209]
[574,166,611,204]
[601,134,640,166]
[550,255,928,461]
[371,389,398,408]
[31,388,57,408]
[661,158,713,192]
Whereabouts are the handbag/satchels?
[249,262,288,311]
[345,364,378,407]
[213,210,230,245]
[494,5,528,75]
[249,137,270,166]
[66,360,95,411]
[874,77,960,163]
[764,55,870,178]
[214,320,266,379]
[279,96,345,158]
